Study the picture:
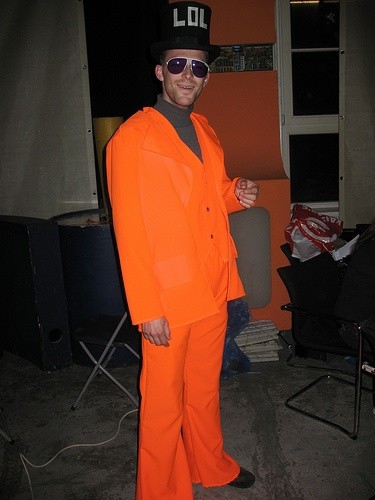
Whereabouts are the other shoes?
[229,467,256,489]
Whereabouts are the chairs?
[71,310,140,412]
[277,223,375,441]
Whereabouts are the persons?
[104,1,261,500]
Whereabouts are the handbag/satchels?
[285,204,344,262]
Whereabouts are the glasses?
[162,56,210,77]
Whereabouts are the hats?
[156,2,220,62]
